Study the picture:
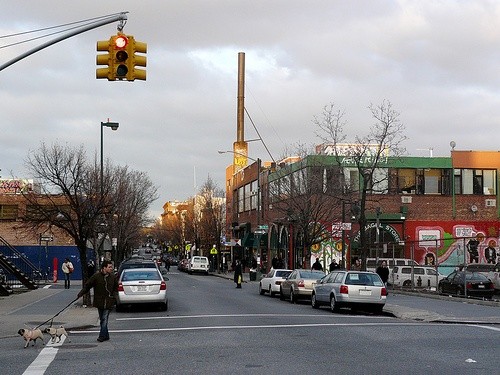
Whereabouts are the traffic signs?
[254,229,267,235]
[41,237,53,241]
[259,226,269,229]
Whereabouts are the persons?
[77,262,119,342]
[376,262,388,295]
[310,258,323,271]
[484,242,500,264]
[250,257,257,270]
[232,260,244,290]
[468,239,479,262]
[164,258,171,271]
[329,260,338,271]
[351,259,361,271]
[61,259,74,289]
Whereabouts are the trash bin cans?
[249,271,257,281]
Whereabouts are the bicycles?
[33,267,57,283]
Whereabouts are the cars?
[311,271,387,313]
[280,269,327,302]
[119,261,159,278]
[259,269,293,296]
[116,268,169,311]
[178,259,188,272]
[438,272,494,299]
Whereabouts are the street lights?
[218,151,259,225]
[101,118,119,269]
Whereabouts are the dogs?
[42,325,72,344]
[18,327,45,348]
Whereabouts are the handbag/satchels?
[238,275,242,284]
[69,268,75,272]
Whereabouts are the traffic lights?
[96,41,111,81]
[132,39,146,80]
[111,35,133,81]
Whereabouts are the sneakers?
[97,336,109,342]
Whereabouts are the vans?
[188,256,209,275]
[366,258,419,276]
[390,266,447,287]
